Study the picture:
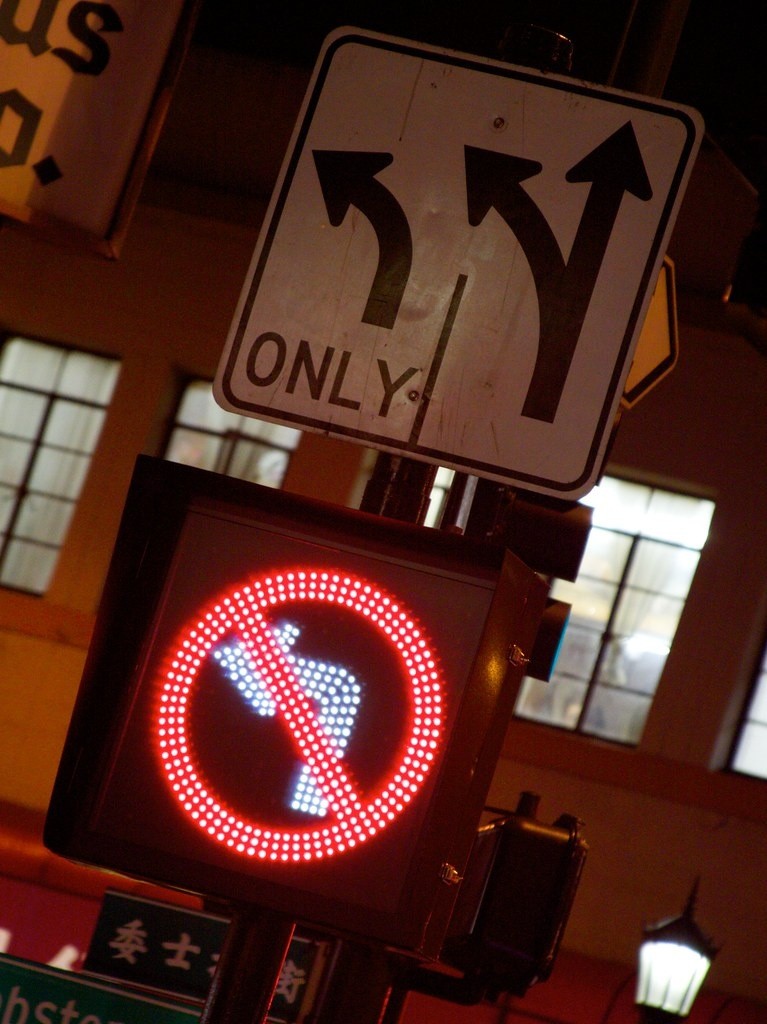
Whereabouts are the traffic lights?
[493,486,595,686]
[440,787,589,986]
[40,450,556,970]
